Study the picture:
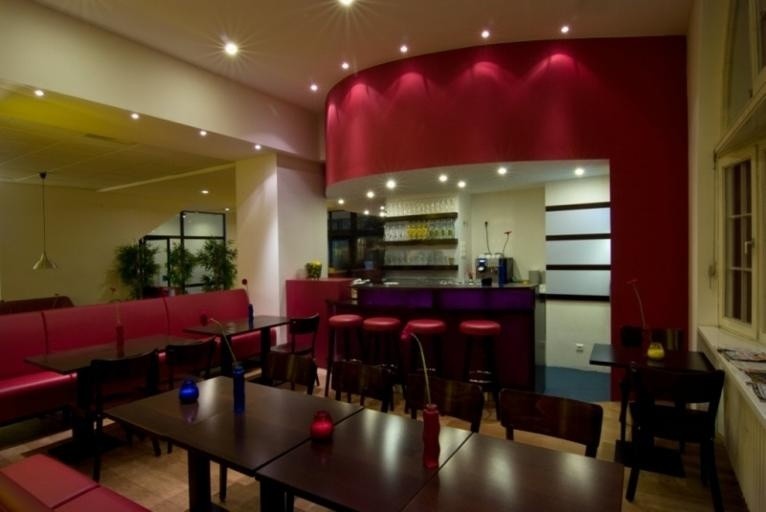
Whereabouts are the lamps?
[31,171,57,271]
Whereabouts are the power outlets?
[482,216,489,224]
[575,344,584,352]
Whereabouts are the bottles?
[646,328,666,361]
[116,325,125,356]
[384,249,448,265]
[385,196,456,216]
[422,403,441,471]
[249,320,254,330]
[233,366,246,414]
[383,218,458,240]
[248,303,254,320]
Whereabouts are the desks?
[23,331,217,483]
[181,317,292,385]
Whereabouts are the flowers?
[501,229,512,258]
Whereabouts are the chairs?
[618,325,687,455]
[624,365,727,512]
[1,454,154,511]
[91,312,321,484]
[1,288,276,428]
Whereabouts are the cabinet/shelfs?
[475,257,513,283]
[697,324,766,512]
[284,277,352,369]
[376,212,458,270]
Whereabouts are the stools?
[323,313,502,424]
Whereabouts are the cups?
[480,270,492,286]
[179,377,199,405]
[309,410,335,443]
[448,256,455,264]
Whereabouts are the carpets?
[614,439,686,478]
